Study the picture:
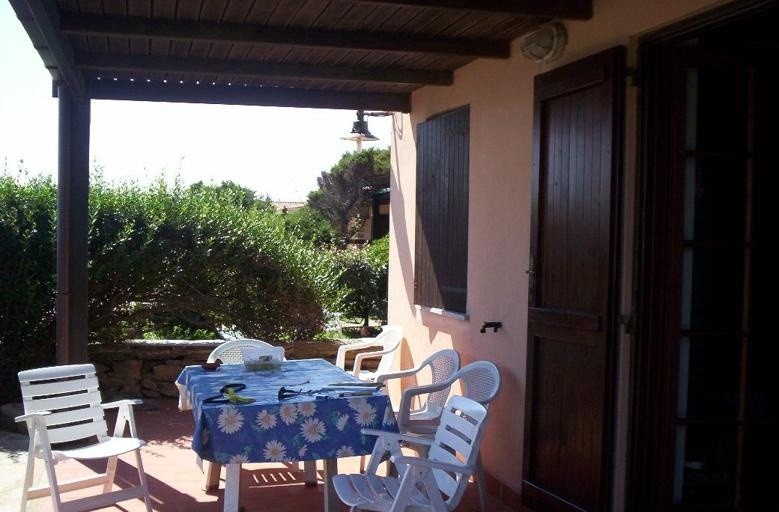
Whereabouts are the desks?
[176,358,398,512]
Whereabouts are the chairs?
[333,325,403,381]
[13,362,153,512]
[332,394,488,511]
[207,339,288,363]
[360,349,459,474]
[397,360,500,512]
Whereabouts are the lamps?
[342,110,397,154]
[520,22,567,64]
